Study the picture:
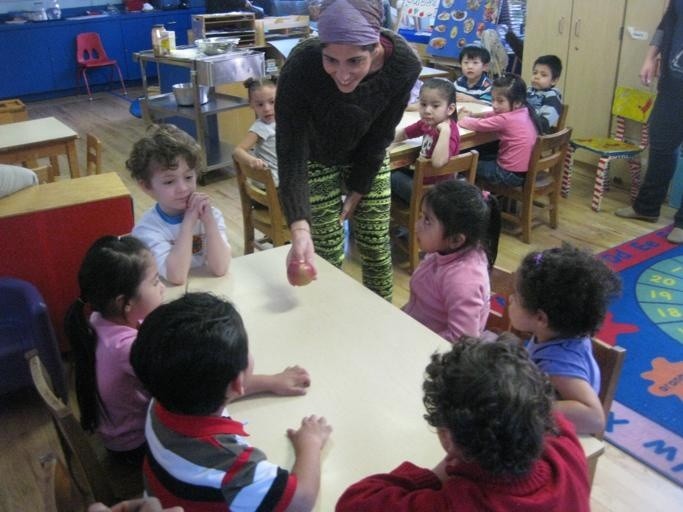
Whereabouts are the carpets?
[475,222,683,486]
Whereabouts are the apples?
[288,263,315,286]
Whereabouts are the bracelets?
[289,226,310,234]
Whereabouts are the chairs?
[556,83,662,215]
[1,95,103,188]
[73,31,128,102]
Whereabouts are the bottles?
[25,1,61,22]
[150,24,170,58]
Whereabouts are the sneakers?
[614,206,658,224]
[665,227,682,244]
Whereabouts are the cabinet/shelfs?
[129,45,264,188]
[1,9,203,100]
[606,1,669,192]
[520,0,624,177]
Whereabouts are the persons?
[396,178,501,341]
[128,294,331,511]
[335,332,593,512]
[387,77,461,217]
[58,235,166,468]
[451,45,495,105]
[228,74,277,209]
[272,0,422,308]
[85,496,183,511]
[125,121,232,286]
[455,72,543,197]
[505,238,607,441]
[612,0,683,245]
[523,55,564,134]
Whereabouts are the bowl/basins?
[194,37,240,56]
[171,83,207,106]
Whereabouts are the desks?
[417,64,449,80]
